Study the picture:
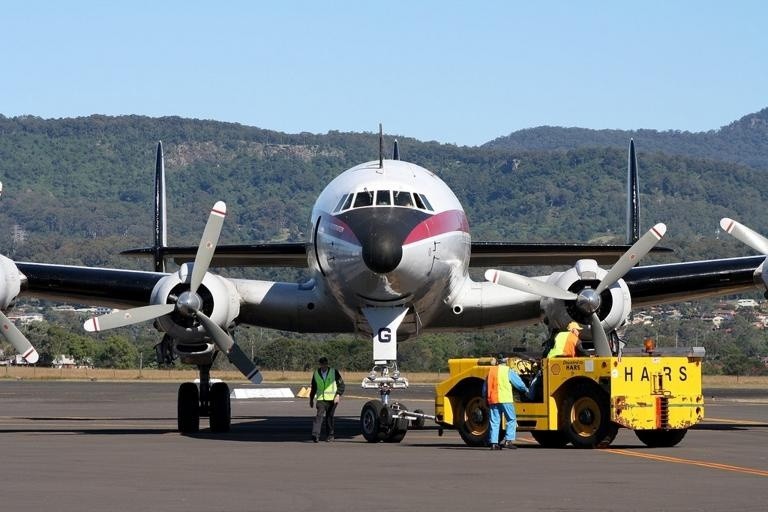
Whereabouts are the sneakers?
[326,436,335,441]
[314,437,320,442]
[492,440,517,450]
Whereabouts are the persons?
[482,354,529,450]
[529,321,588,400]
[310,357,345,443]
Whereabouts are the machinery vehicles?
[436,339,706,448]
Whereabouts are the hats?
[319,357,328,364]
[567,321,584,330]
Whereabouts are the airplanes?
[0,122,768,442]
